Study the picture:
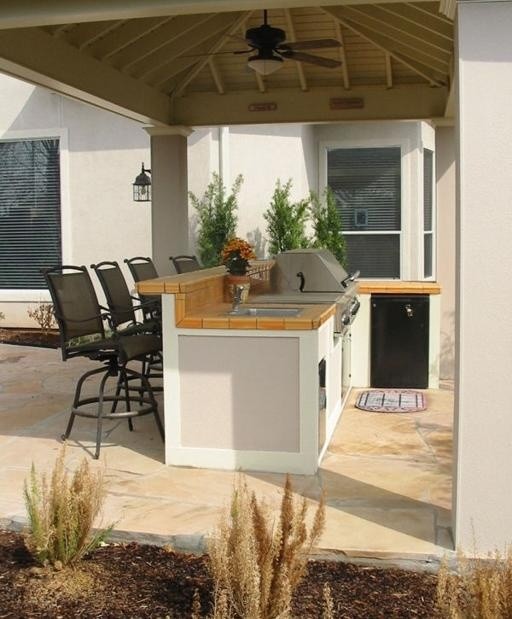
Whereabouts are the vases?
[225,264,250,304]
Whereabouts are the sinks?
[225,306,306,318]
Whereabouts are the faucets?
[231,283,245,309]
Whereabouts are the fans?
[176,9,343,69]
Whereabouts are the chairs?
[39,255,203,460]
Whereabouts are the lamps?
[248,48,284,76]
[132,162,151,202]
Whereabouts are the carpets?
[354,389,427,414]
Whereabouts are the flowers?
[221,237,256,268]
